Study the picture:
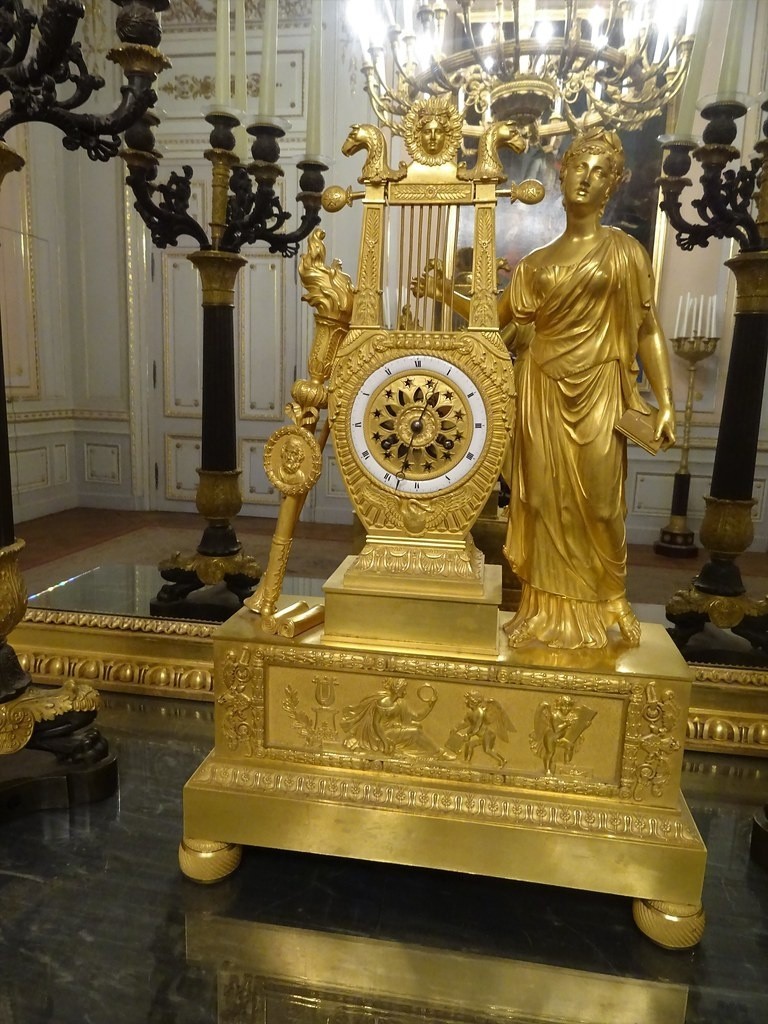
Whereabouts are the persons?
[275,437,306,485]
[454,688,511,768]
[424,121,444,154]
[409,128,679,646]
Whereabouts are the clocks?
[350,356,487,493]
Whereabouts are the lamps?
[342,0,701,155]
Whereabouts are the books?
[617,401,668,456]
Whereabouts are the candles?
[672,296,682,339]
[683,291,717,340]
[214,0,232,106]
[235,0,248,110]
[257,0,279,115]
